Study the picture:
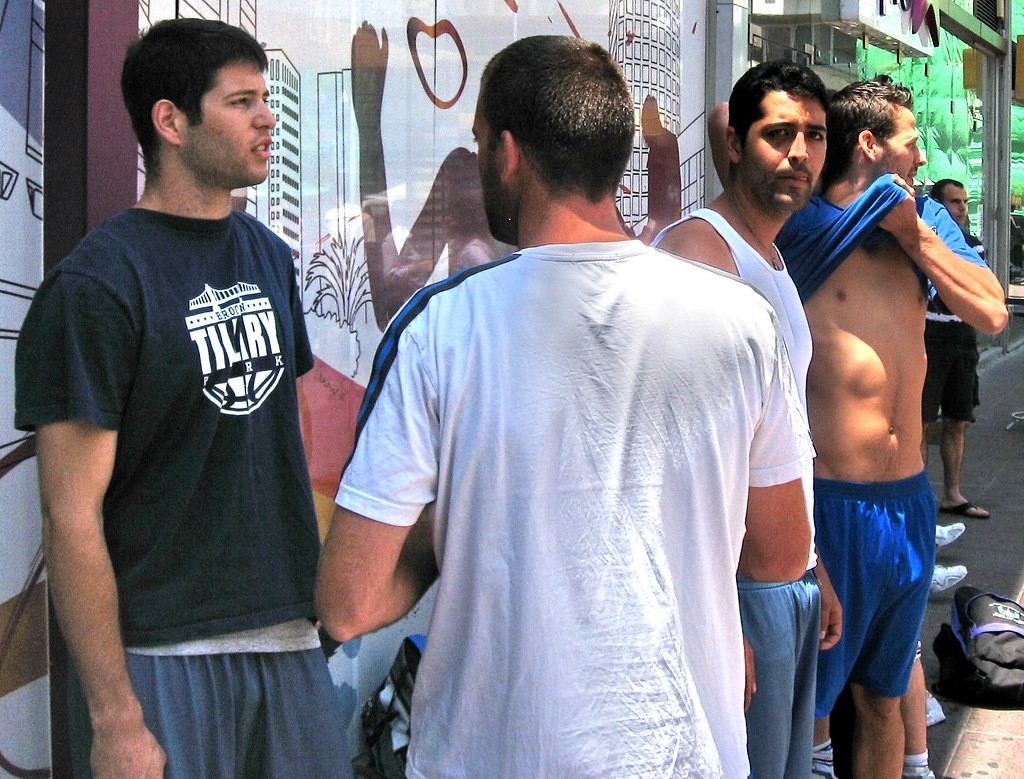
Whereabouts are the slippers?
[938,502,990,519]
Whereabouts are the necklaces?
[745,223,780,270]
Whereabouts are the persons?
[311,36,818,779]
[13,18,354,779]
[653,58,1011,779]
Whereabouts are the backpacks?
[934,586,1024,699]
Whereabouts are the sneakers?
[935,522,965,548]
[928,563,969,592]
